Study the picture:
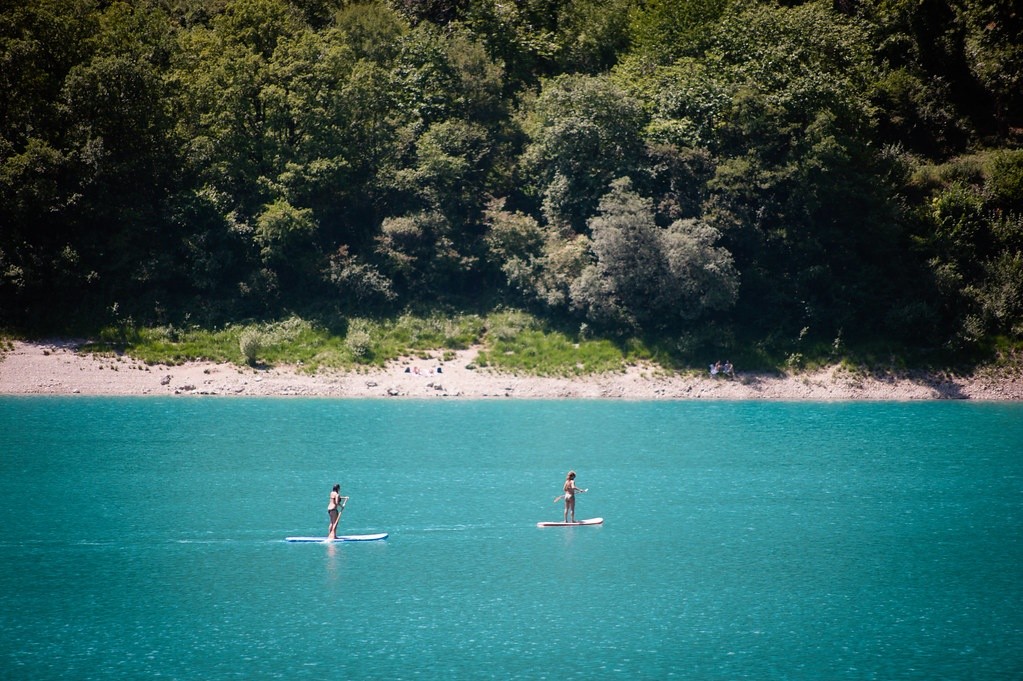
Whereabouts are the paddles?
[554,488,589,503]
[327,498,347,539]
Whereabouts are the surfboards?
[285,532,388,541]
[537,518,603,526]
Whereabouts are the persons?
[327,484,343,539]
[710,360,734,381]
[563,471,583,523]
